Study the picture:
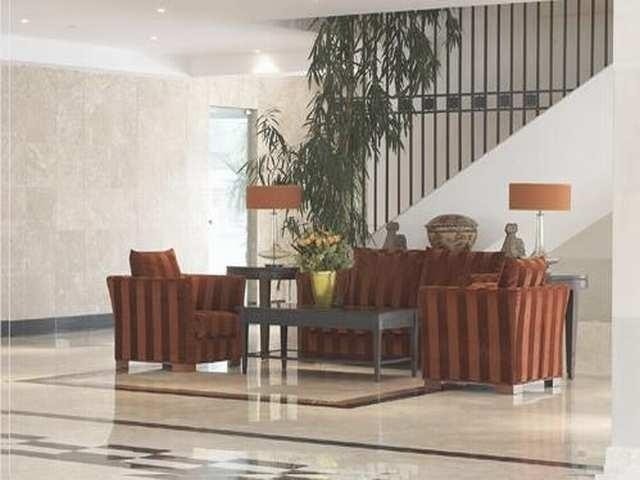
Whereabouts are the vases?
[311,271,336,308]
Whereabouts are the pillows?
[129,248,182,276]
[497,255,549,287]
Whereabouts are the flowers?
[292,224,354,271]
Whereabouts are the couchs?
[298,248,505,361]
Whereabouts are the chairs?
[106,275,244,373]
[418,283,571,395]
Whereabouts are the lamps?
[247,186,301,268]
[510,184,571,274]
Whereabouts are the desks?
[227,265,300,356]
[548,274,590,379]
